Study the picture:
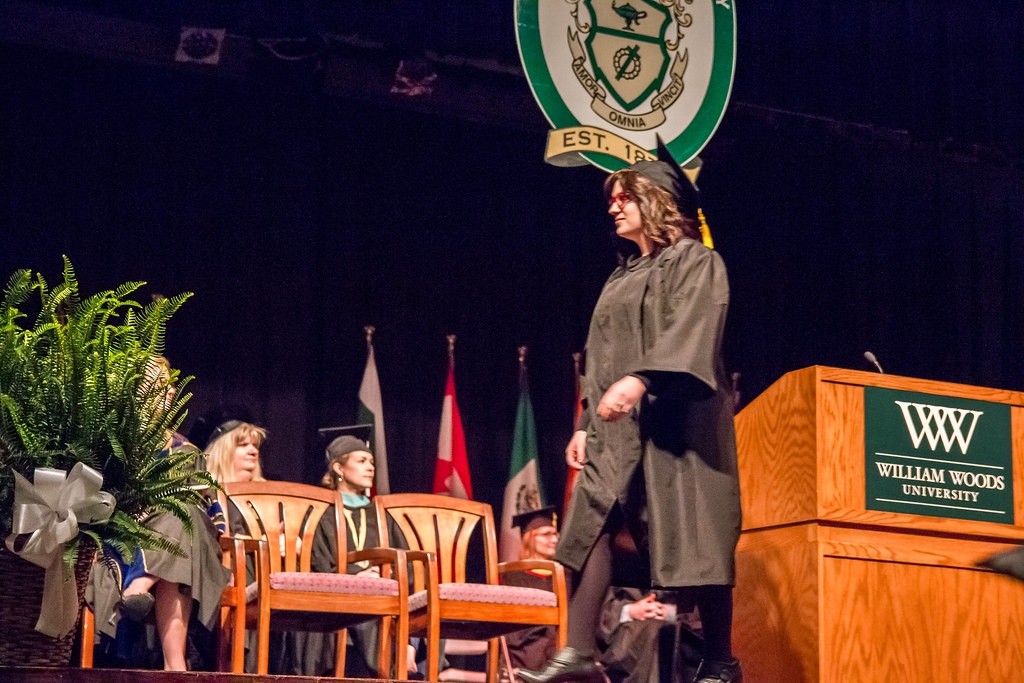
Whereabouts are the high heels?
[108,593,155,627]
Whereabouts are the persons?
[513,159,743,683]
[298,436,448,683]
[500,504,569,672]
[595,584,710,683]
[130,358,341,683]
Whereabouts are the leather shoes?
[694,657,741,683]
[514,647,602,683]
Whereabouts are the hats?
[510,506,558,534]
[188,403,255,447]
[629,133,715,249]
[319,422,375,459]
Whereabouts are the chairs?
[80,482,569,683]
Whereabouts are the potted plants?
[0,253,217,668]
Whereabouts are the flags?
[359,343,391,500]
[561,373,589,528]
[434,358,477,586]
[500,363,545,587]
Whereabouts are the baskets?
[0,542,100,668]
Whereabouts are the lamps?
[176,27,225,65]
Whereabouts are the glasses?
[608,192,635,205]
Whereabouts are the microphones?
[864,351,884,374]
